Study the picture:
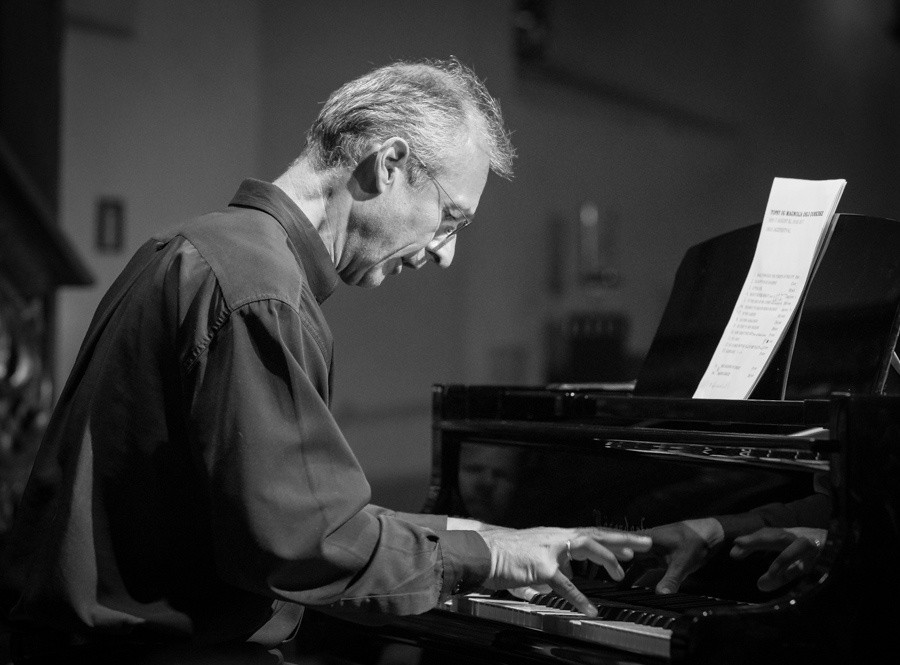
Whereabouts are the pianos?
[420,213,899,664]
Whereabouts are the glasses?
[408,146,474,239]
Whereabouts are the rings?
[566,538,574,562]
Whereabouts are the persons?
[630,469,836,594]
[0,52,656,664]
